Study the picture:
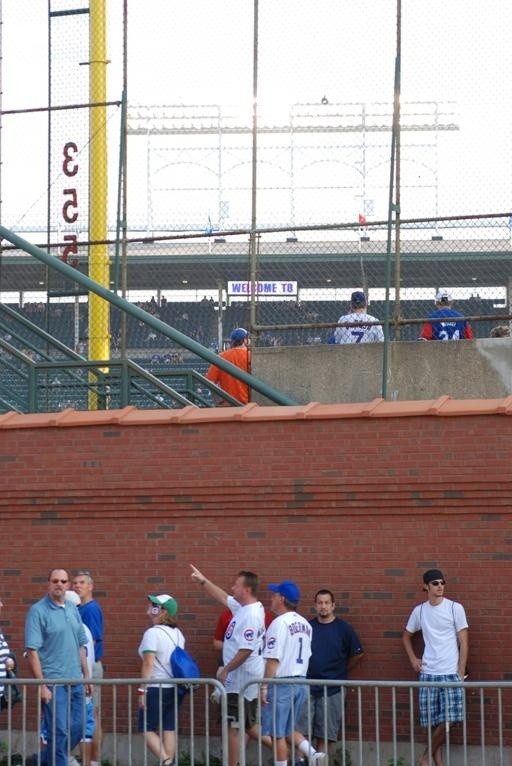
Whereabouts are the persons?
[213,607,274,749]
[403,570,468,766]
[327,291,385,344]
[0,601,18,715]
[206,328,251,407]
[418,290,474,341]
[135,594,186,766]
[72,571,103,766]
[24,568,92,766]
[260,582,328,766]
[191,564,272,766]
[469,293,481,303]
[1,292,241,414]
[63,590,95,766]
[295,590,364,766]
[489,325,511,338]
[258,299,325,346]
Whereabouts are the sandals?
[163,758,173,766]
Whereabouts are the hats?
[268,580,299,605]
[147,594,178,617]
[351,292,364,304]
[436,292,453,303]
[231,328,249,340]
[422,569,443,592]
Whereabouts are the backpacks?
[154,627,201,693]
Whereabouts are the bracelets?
[137,688,148,695]
[200,578,207,585]
[261,684,269,691]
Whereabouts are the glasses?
[431,580,445,585]
[152,602,158,607]
[52,579,67,584]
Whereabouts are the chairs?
[0,358,243,413]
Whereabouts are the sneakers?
[311,752,328,766]
